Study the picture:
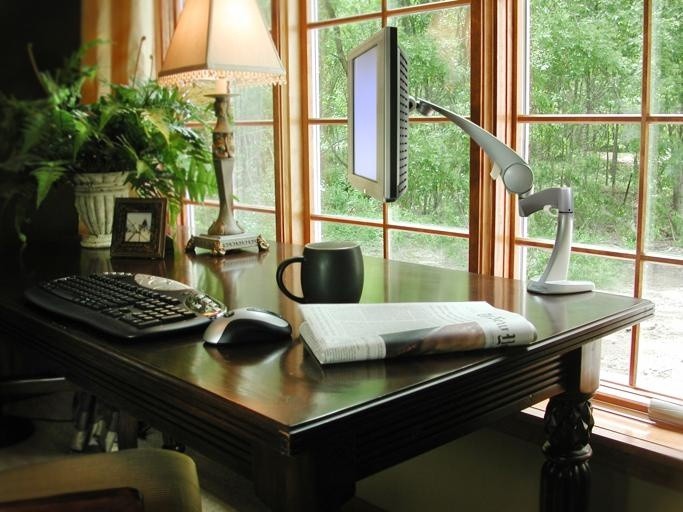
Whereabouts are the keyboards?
[24,269,228,340]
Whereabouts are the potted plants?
[1,38,234,250]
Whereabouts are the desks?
[5,239,626,510]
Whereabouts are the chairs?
[2,375,203,509]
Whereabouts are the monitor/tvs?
[347,25,595,293]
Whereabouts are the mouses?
[204,305,291,347]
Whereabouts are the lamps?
[156,0,287,255]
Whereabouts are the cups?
[276,240,366,305]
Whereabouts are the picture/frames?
[112,198,165,261]
